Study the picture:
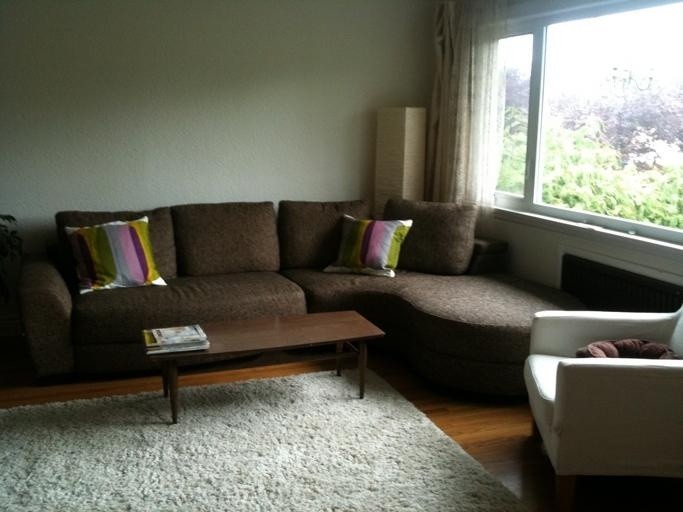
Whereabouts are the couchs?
[20,196,587,412]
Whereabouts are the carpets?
[0,362,528,511]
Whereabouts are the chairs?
[522,303,682,484]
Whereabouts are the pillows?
[277,196,371,273]
[52,203,177,284]
[380,197,479,276]
[322,211,415,279]
[574,335,681,361]
[62,214,168,295]
[171,198,281,275]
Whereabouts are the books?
[140,323,210,355]
